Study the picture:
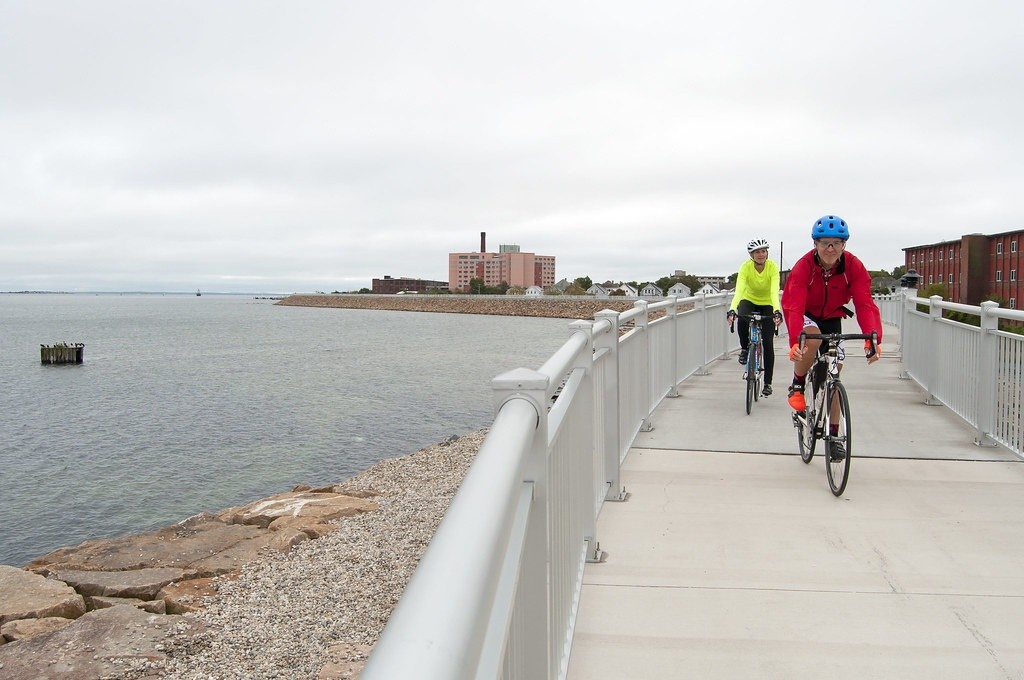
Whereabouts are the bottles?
[815,385,824,411]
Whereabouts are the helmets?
[812,215,849,240]
[747,239,769,253]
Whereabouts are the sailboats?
[196,289,201,296]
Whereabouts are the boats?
[568,318,633,329]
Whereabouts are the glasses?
[816,240,846,251]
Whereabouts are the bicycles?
[790,330,879,497]
[729,310,779,415]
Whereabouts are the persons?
[781,215,882,464]
[726,239,782,396]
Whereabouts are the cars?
[397,291,406,295]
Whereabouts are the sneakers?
[788,386,806,411]
[830,442,846,459]
[762,385,772,395]
[739,349,748,364]
